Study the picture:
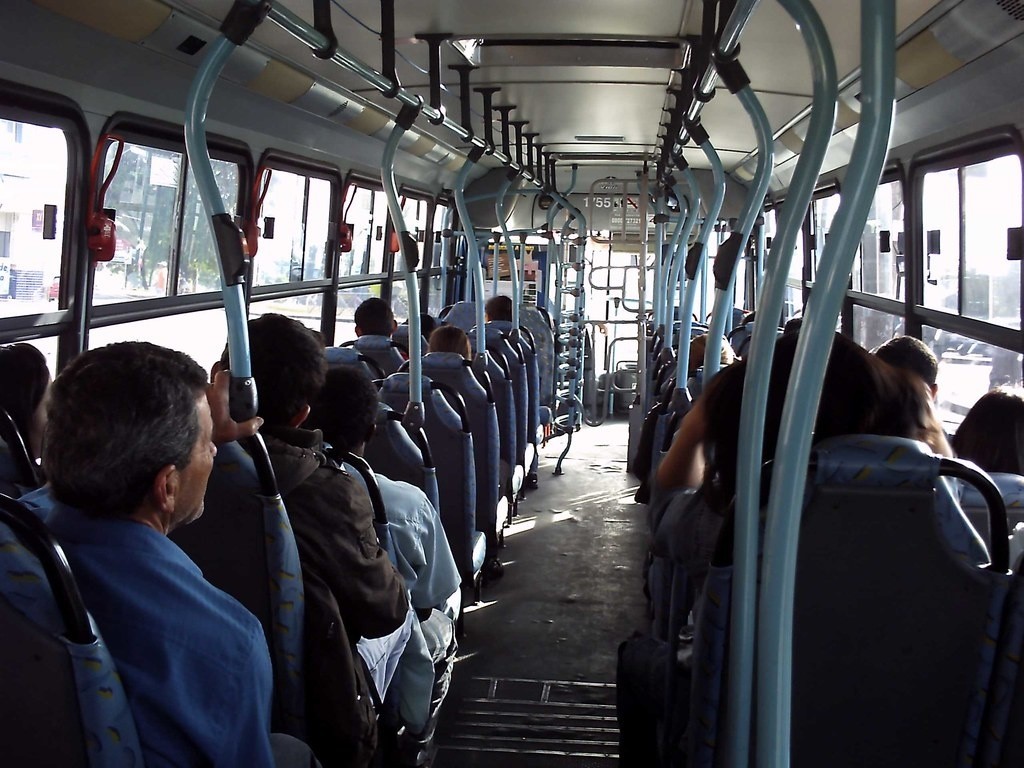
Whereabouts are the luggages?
[618,551,691,768]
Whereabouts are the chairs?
[1,301,556,768]
[641,304,1024,768]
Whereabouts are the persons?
[1,295,553,767]
[157,261,168,295]
[632,312,1024,618]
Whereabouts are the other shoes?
[488,557,504,580]
